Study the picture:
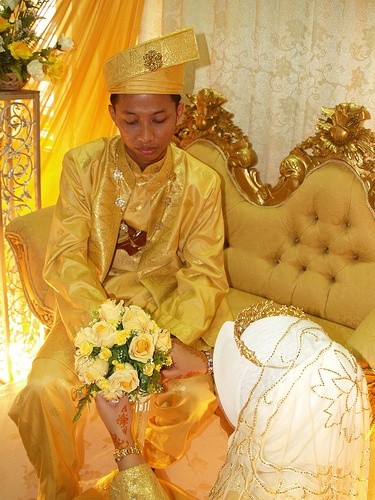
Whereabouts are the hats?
[102,26,200,97]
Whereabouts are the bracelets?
[114,443,139,462]
[201,350,214,375]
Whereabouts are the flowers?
[72,298,172,427]
[0,0,76,88]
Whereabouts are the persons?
[7,26,236,500]
[95,314,375,500]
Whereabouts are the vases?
[1,73,33,88]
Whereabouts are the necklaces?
[113,143,177,251]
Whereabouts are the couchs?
[5,89,375,369]
